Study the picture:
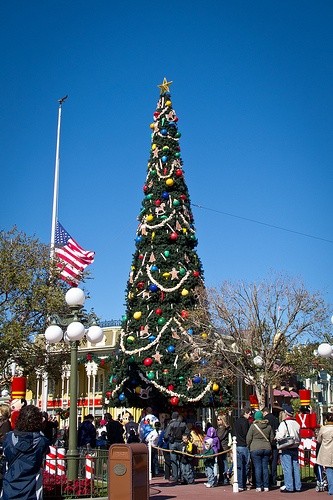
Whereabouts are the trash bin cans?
[108,444,149,500]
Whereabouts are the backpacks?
[205,449,214,460]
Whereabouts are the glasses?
[217,419,221,421]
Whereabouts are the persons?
[77,414,96,448]
[125,415,139,437]
[102,412,125,445]
[232,407,252,491]
[138,407,162,475]
[261,407,280,485]
[187,423,204,441]
[316,413,333,495]
[198,427,220,487]
[216,414,232,485]
[246,411,274,492]
[0,404,12,438]
[158,412,195,484]
[275,407,302,493]
[296,389,321,468]
[40,412,57,446]
[0,405,51,500]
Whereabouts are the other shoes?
[263,487,270,492]
[203,482,219,488]
[256,487,262,492]
[280,488,294,493]
[296,489,302,492]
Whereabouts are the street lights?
[45,287,105,483]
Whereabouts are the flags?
[54,221,95,287]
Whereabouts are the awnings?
[273,389,299,397]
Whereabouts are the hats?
[254,411,263,420]
[282,405,294,417]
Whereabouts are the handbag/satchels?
[168,432,176,443]
[276,436,295,450]
